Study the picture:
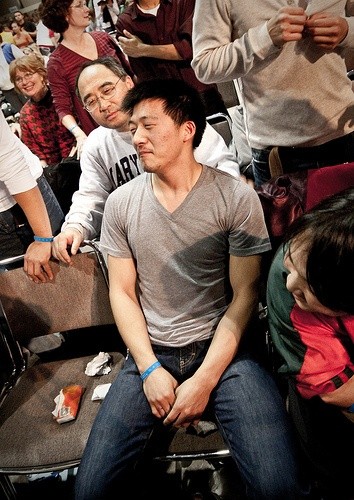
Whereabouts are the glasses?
[83,78,121,112]
[69,0,87,9]
[15,71,36,85]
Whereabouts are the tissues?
[51,383,84,425]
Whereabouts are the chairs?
[0,238,126,500]
[149,416,249,500]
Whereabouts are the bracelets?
[32,234,55,243]
[139,360,161,379]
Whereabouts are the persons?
[190,0,353,185]
[265,186,354,423]
[73,73,304,500]
[38,0,138,160]
[9,53,78,164]
[50,57,241,267]
[115,0,232,123]
[0,0,120,100]
[0,106,64,282]
